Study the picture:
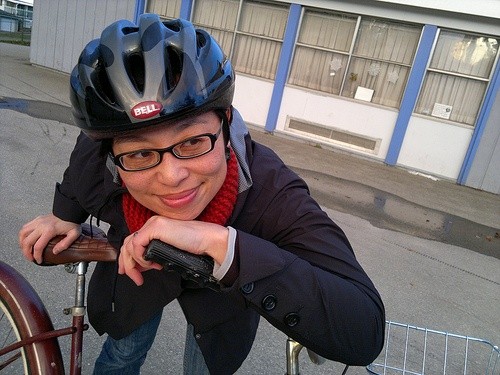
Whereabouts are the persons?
[18,13,386,374]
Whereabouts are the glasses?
[109,117,225,172]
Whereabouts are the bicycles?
[1,223,500,374]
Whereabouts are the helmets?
[69,15,239,141]
